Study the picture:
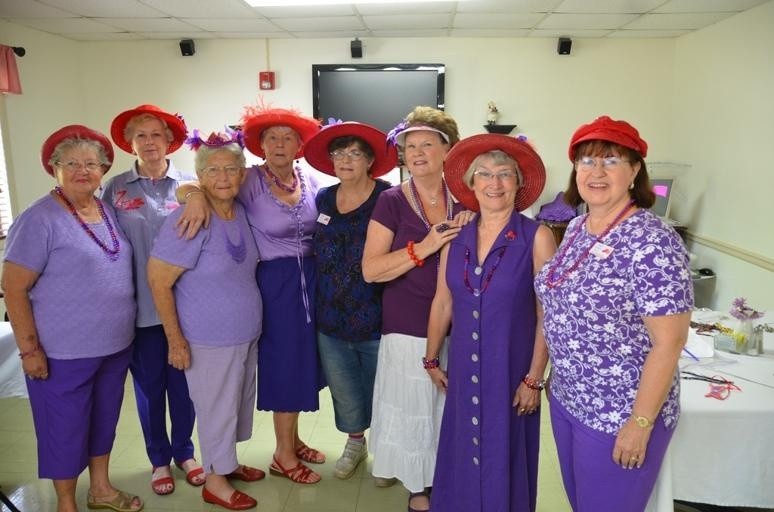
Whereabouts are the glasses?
[472,167,517,178]
[330,147,368,161]
[577,156,629,169]
[203,164,244,178]
[55,159,102,171]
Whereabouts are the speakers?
[351,41,362,58]
[180,39,195,56]
[557,37,572,55]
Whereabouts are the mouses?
[698,267,712,276]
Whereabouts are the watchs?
[630,413,653,431]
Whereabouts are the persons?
[303,118,399,488]
[1,125,146,512]
[97,105,206,495]
[147,128,265,510]
[533,116,696,512]
[176,91,325,484]
[362,106,478,512]
[422,133,560,511]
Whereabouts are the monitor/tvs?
[312,63,446,135]
[649,176,673,219]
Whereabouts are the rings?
[28,374,34,379]
[520,407,526,414]
[182,219,190,224]
[528,408,535,415]
[631,456,638,462]
[436,223,450,232]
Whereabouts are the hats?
[396,118,450,148]
[111,105,186,154]
[569,116,647,164]
[42,125,113,177]
[197,130,238,146]
[444,134,545,212]
[244,109,320,159]
[305,122,397,179]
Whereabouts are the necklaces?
[264,161,298,193]
[53,185,121,262]
[407,175,452,232]
[463,227,518,295]
[545,199,634,288]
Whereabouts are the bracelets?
[184,185,203,202]
[407,240,425,266]
[521,375,546,392]
[20,345,42,360]
[423,357,440,368]
